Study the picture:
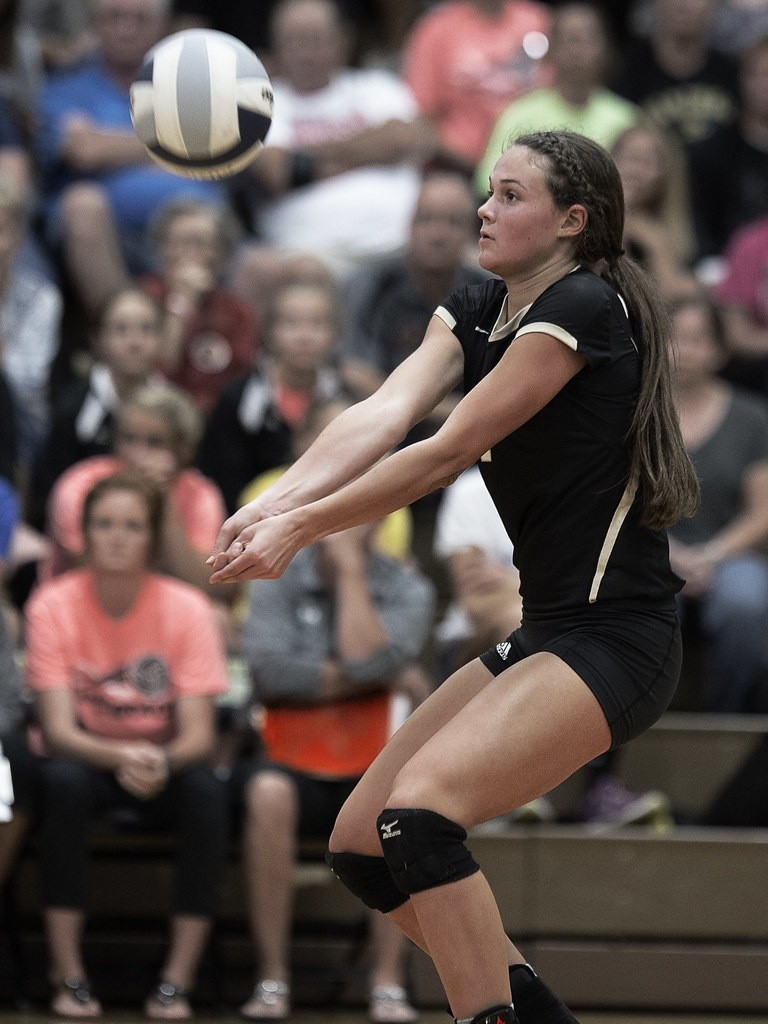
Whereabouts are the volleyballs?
[127,26,276,182]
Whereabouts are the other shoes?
[369,982,419,1024]
[48,974,104,1019]
[240,982,294,1022]
[146,979,194,1019]
[448,964,582,1024]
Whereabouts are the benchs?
[0,712,768,1024]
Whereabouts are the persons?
[0,0,768,1024]
[20,471,233,1018]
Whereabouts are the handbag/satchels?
[245,688,413,784]
